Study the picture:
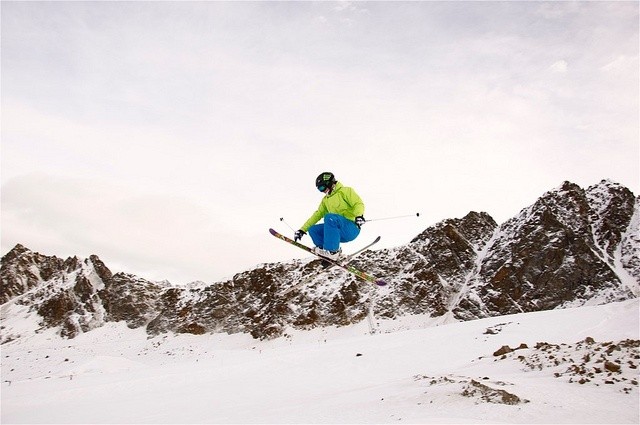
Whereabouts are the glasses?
[318,175,336,193]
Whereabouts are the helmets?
[316,172,339,196]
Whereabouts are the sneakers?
[317,250,341,262]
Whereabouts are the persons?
[295,172,366,262]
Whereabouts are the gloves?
[294,230,309,243]
[355,216,367,230]
